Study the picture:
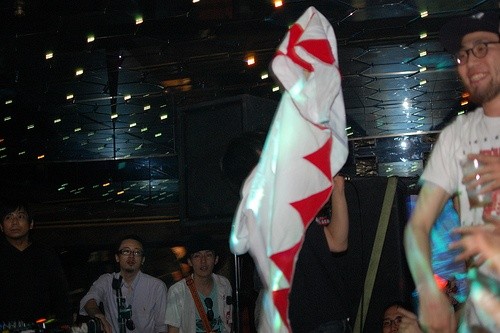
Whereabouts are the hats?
[179,240,217,264]
[439,9,500,54]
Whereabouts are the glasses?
[191,253,214,258]
[382,317,402,327]
[118,247,144,256]
[453,41,500,64]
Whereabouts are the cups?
[459,157,493,207]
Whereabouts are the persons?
[289,17,500,333]
[0,201,233,333]
